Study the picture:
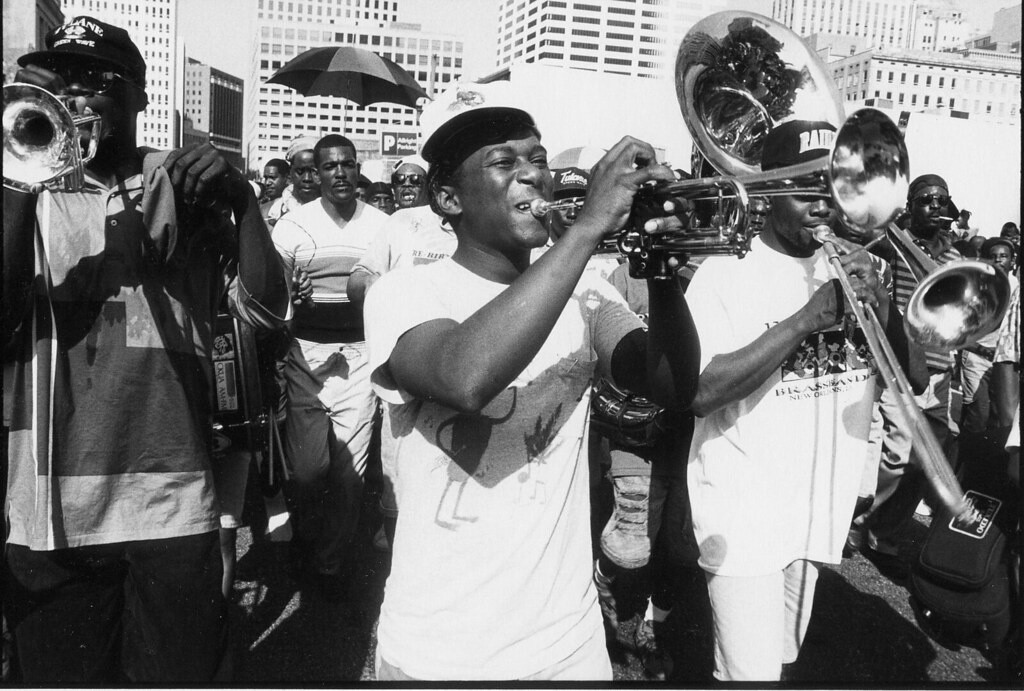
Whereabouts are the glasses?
[912,192,951,205]
[391,173,427,184]
[45,62,142,95]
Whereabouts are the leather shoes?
[867,529,899,556]
[846,522,866,551]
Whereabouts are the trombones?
[813,224,1011,526]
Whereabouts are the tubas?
[672,8,840,179]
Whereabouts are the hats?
[419,79,538,162]
[552,166,590,193]
[16,15,146,87]
[391,154,431,175]
[761,119,839,170]
[286,136,321,160]
[906,174,949,201]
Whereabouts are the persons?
[0,17,1023,681]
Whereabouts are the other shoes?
[364,511,390,551]
[595,570,616,619]
[634,620,674,680]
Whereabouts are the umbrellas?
[264,47,434,137]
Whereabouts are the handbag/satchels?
[910,464,1013,645]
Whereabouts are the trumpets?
[530,106,911,282]
[2,83,103,194]
